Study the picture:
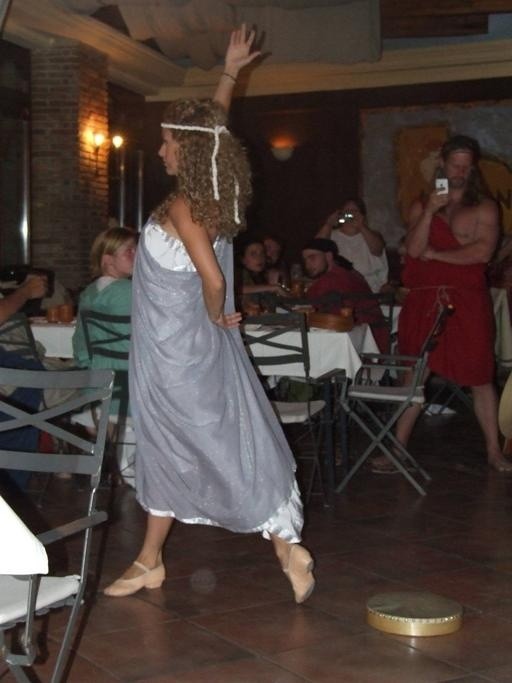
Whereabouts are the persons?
[369,134,512,474]
[0,271,54,517]
[235,199,392,469]
[71,226,142,508]
[101,21,315,605]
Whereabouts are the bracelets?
[16,287,27,304]
[221,71,239,84]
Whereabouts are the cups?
[278,270,290,292]
[48,307,58,323]
[59,303,73,322]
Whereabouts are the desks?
[28,311,380,491]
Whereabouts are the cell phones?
[337,211,354,224]
[435,177,449,195]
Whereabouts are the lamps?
[106,135,123,149]
[94,133,103,150]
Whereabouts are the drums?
[366,591,464,636]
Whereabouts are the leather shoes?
[281,544,316,604]
[103,561,166,597]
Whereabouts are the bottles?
[291,265,304,298]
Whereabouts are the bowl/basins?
[10,271,27,284]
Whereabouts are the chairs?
[379,292,395,333]
[0,365,118,683]
[323,301,456,507]
[78,308,127,484]
[240,312,346,511]
[0,318,68,459]
